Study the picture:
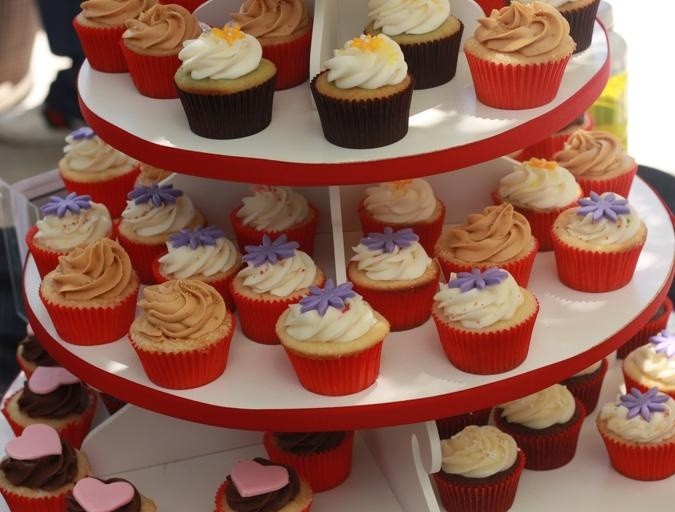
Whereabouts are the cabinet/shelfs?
[1,0,675,512]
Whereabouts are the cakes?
[261,430,355,495]
[72,0,313,139]
[0,332,163,512]
[215,458,313,512]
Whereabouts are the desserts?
[25,127,647,398]
[309,0,601,146]
[432,295,674,512]
